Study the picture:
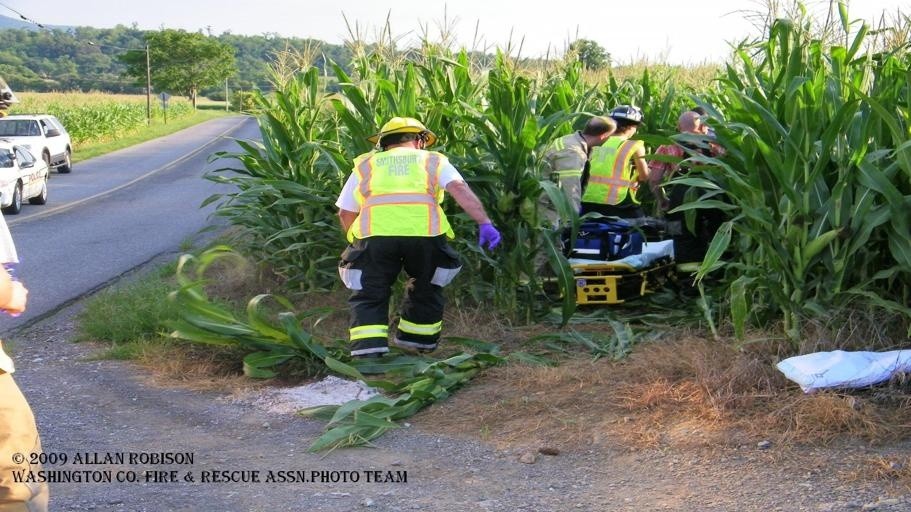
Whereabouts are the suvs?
[1,114,75,179]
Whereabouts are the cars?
[0,139,53,213]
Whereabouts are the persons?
[519,106,725,295]
[336,116,502,377]
[0,74,52,512]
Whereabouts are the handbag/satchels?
[559,218,642,261]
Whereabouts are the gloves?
[479,224,501,250]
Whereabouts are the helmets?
[610,106,644,124]
[366,117,437,148]
